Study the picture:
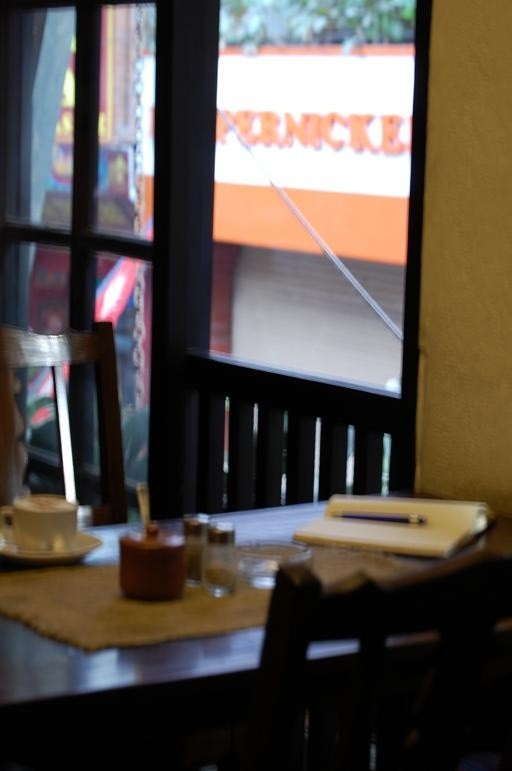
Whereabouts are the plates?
[0,531,101,567]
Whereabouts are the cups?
[0,496,79,551]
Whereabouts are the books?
[290,491,498,560]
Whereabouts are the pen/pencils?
[328,510,423,525]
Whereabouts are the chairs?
[0,320,139,524]
[262,545,508,767]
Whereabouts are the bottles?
[180,517,235,598]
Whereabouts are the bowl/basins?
[235,539,315,587]
